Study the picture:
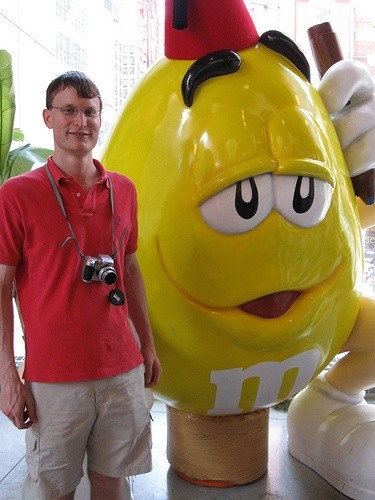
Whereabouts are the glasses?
[47,106,102,118]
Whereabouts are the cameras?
[81,254,118,285]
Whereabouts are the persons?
[1,68,161,500]
